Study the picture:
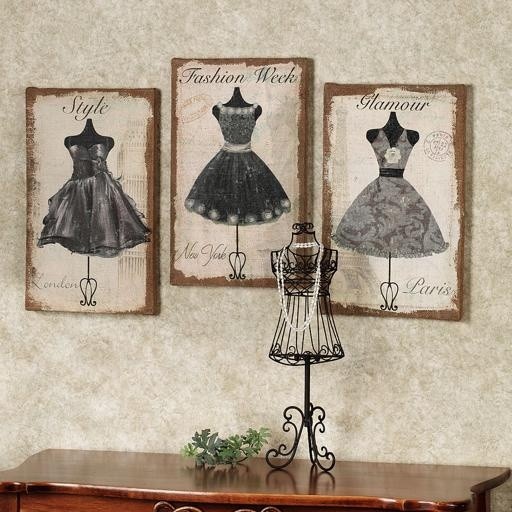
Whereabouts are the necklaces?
[276,243,325,330]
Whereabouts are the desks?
[0,449,510,512]
